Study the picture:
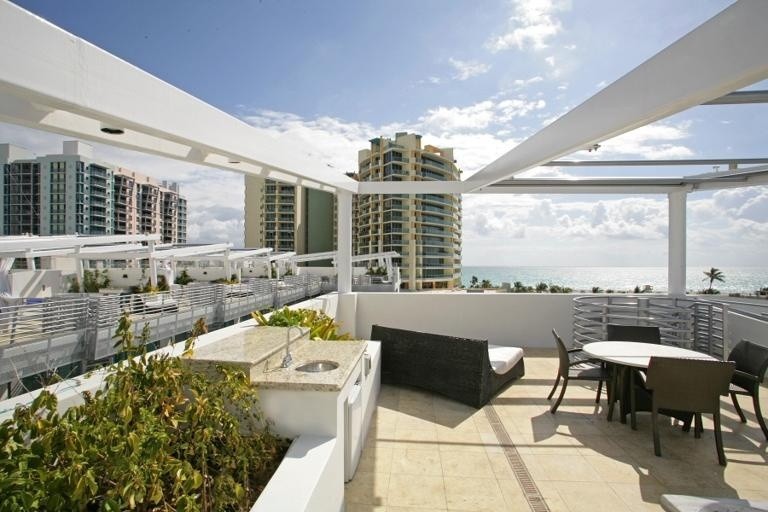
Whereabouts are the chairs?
[371,277,392,285]
[606,322,661,406]
[547,327,618,422]
[694,337,768,442]
[117,292,179,315]
[629,355,737,467]
[228,290,254,298]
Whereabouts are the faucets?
[281,323,304,367]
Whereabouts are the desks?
[659,493,768,512]
[582,341,721,432]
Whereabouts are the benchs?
[370,323,526,409]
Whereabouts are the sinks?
[296,360,339,373]
